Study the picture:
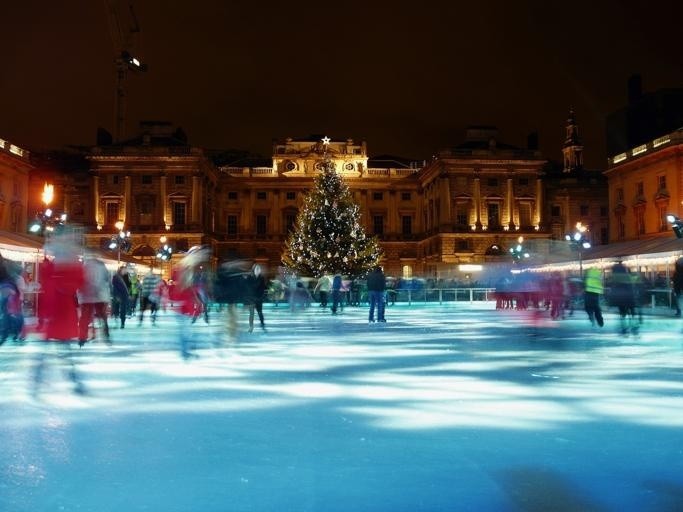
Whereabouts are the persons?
[0,238,394,399]
[492,255,682,343]
